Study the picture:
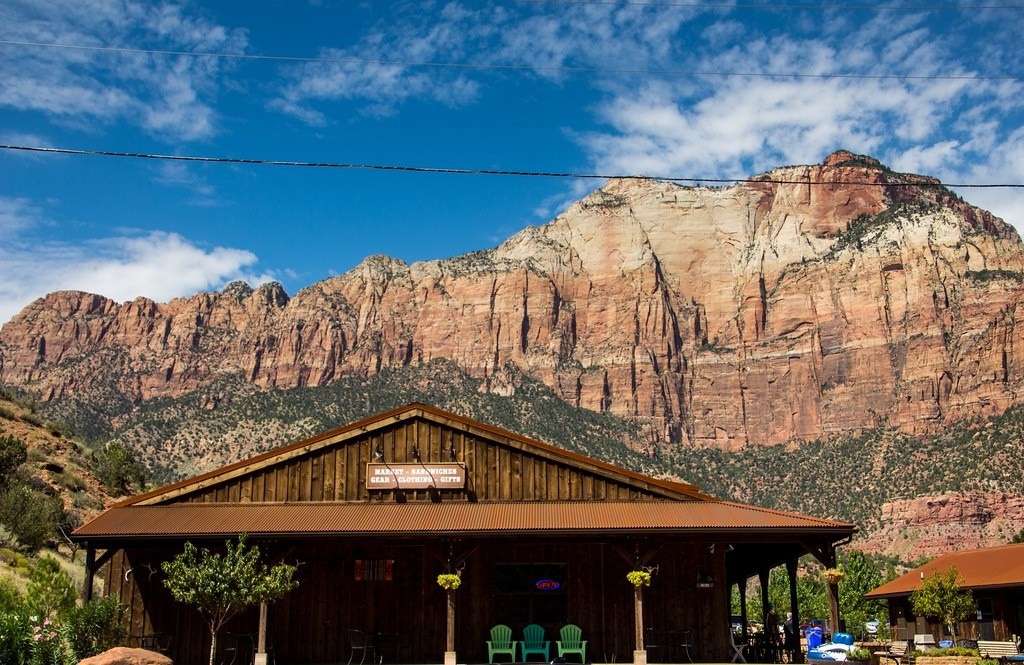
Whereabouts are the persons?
[783,613,794,663]
[767,603,785,663]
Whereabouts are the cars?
[731,613,753,646]
[866,615,879,634]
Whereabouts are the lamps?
[373,442,454,460]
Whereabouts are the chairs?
[347,628,415,665]
[729,628,748,663]
[220,630,276,665]
[555,623,588,664]
[135,634,172,655]
[485,624,518,664]
[519,623,552,664]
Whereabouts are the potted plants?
[823,567,846,584]
[627,570,651,586]
[436,573,462,590]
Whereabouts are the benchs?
[874,640,909,665]
[977,640,1024,665]
